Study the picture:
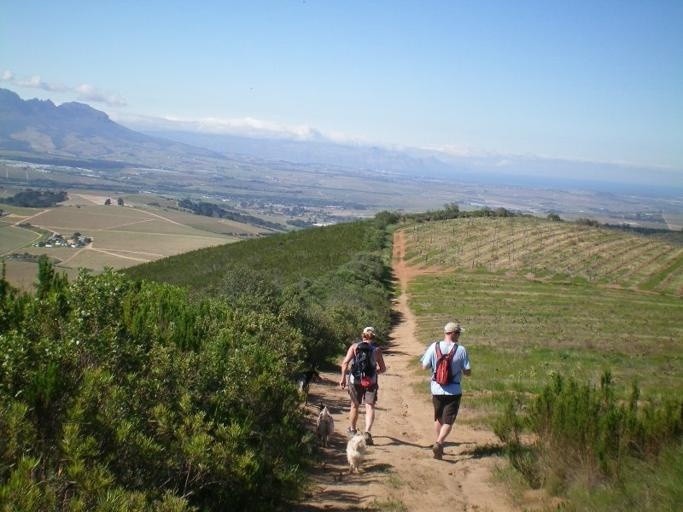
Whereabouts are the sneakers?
[432,442,443,460]
[364,432,373,445]
[346,427,356,435]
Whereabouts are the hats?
[362,327,376,336]
[444,322,466,334]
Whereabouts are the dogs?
[313,401,336,450]
[346,429,376,476]
[292,365,324,405]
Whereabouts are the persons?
[340,326,386,445]
[420,322,471,459]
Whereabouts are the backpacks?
[349,342,380,379]
[432,342,460,386]
